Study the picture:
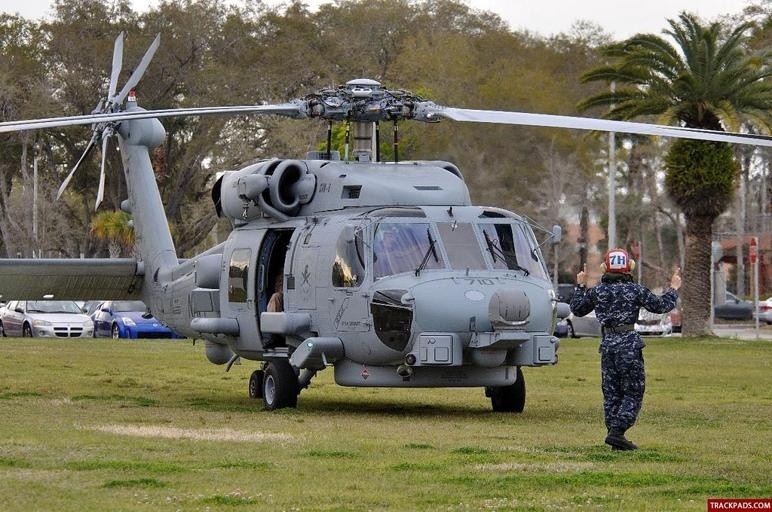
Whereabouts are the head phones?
[600,248,636,273]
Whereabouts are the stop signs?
[749,235,759,264]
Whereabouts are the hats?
[605,248,630,274]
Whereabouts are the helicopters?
[0,29,772,413]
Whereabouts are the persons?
[570,246,683,450]
[374,226,401,279]
[260,266,284,349]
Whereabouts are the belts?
[603,324,635,336]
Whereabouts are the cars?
[552,278,772,343]
[0,301,183,338]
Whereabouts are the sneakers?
[606,427,638,451]
